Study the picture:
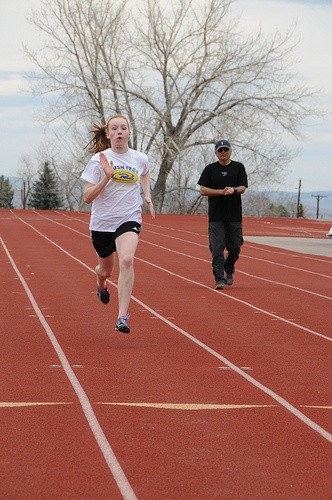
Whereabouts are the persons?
[197,140,249,289]
[79,114,155,334]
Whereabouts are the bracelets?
[233,187,237,194]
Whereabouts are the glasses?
[217,147,228,152]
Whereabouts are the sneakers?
[96,284,110,304]
[225,270,234,284]
[115,315,130,333]
[214,281,225,289]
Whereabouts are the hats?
[214,140,231,151]
